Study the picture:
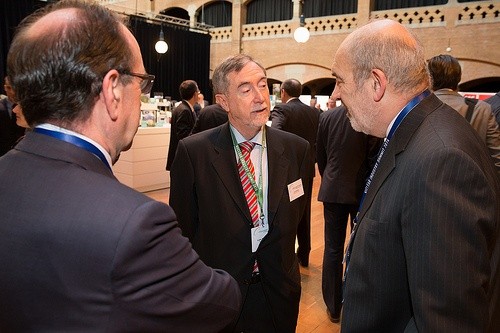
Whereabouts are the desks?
[113,127,171,193]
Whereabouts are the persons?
[316,100,384,323]
[192,104,229,134]
[310,96,338,113]
[166,80,200,207]
[330,18,500,333]
[170,53,314,333]
[12,102,32,135]
[0,76,26,157]
[196,92,211,108]
[0,0,244,333]
[429,53,500,175]
[484,89,500,127]
[271,79,319,268]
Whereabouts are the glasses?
[118,69,156,96]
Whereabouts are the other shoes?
[326,306,340,323]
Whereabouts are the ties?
[236,141,261,279]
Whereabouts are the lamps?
[155,30,168,53]
[294,15,310,43]
